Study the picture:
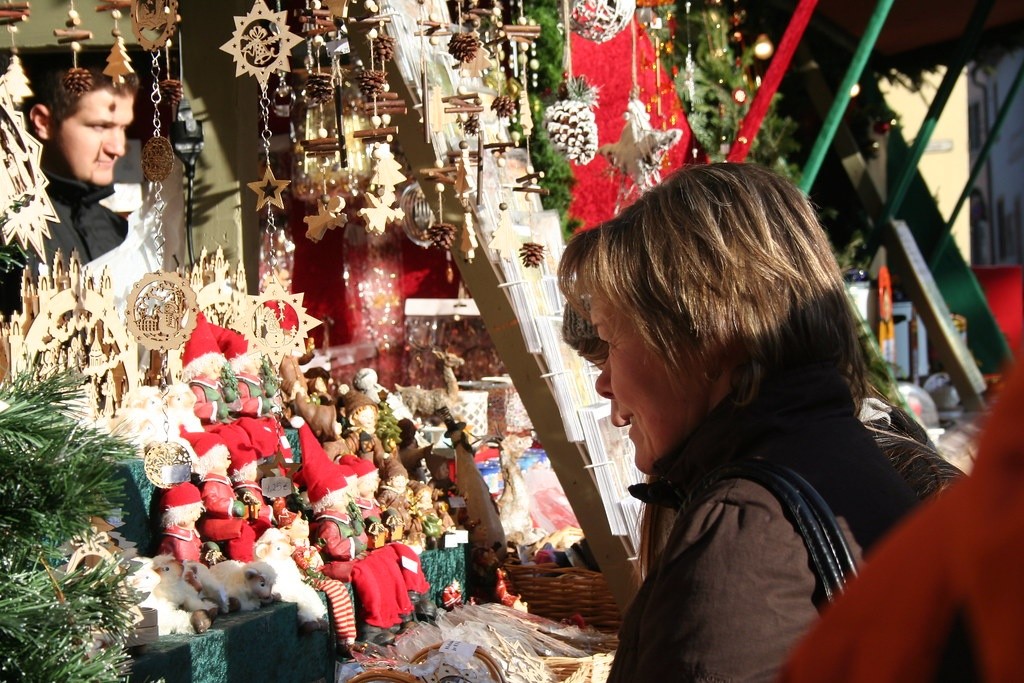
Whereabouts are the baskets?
[408,625,617,683]
[501,554,624,634]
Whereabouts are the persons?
[159,481,226,570]
[178,423,256,562]
[558,160,1023,683]
[205,310,469,648]
[1,52,141,322]
[180,311,241,433]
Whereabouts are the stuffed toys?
[129,551,278,635]
[99,383,205,458]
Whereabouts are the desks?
[116,538,466,683]
